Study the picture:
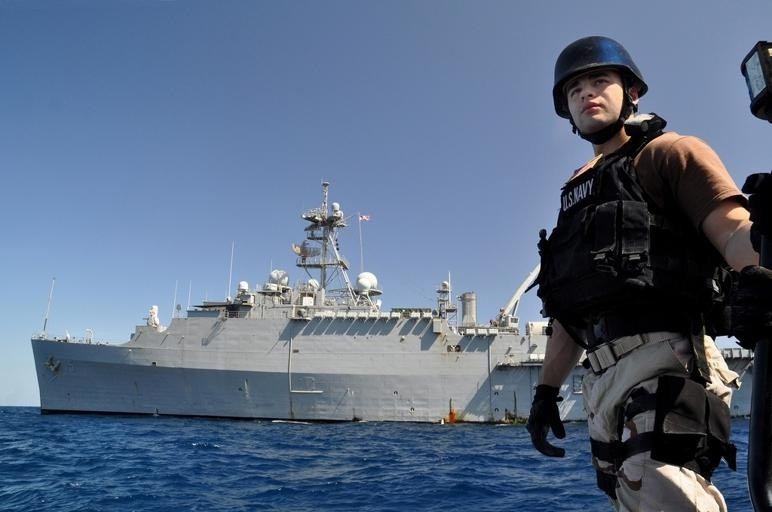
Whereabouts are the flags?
[359,215,370,221]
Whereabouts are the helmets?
[552,35,648,119]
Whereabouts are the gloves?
[525,384,566,457]
[736,264,772,323]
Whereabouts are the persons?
[522,35,770,512]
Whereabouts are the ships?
[28,179,753,425]
